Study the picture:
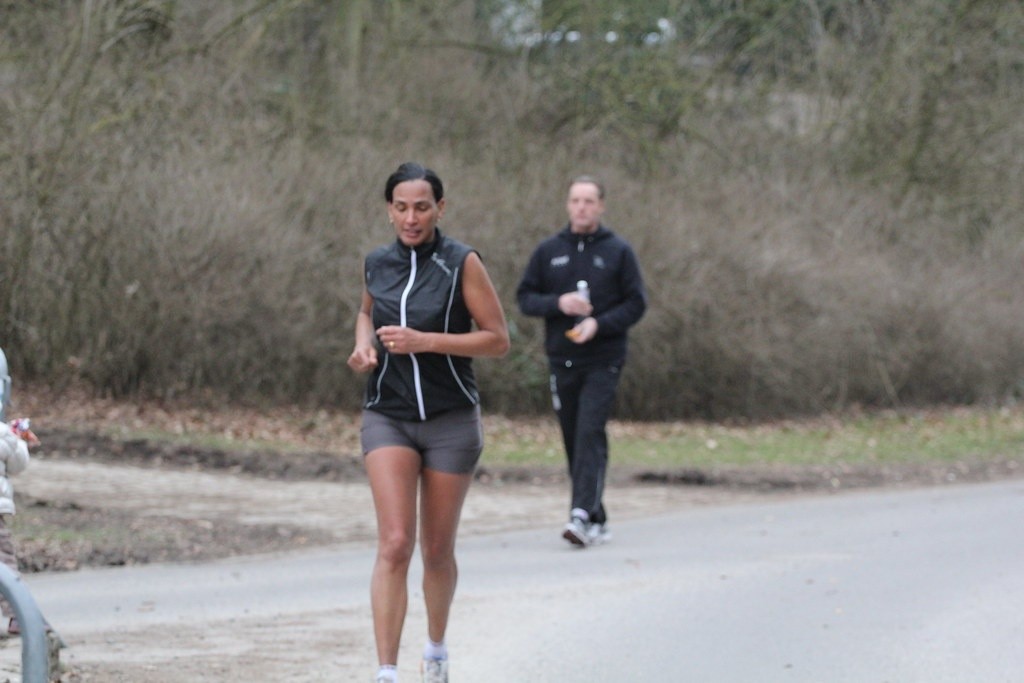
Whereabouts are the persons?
[348,163,510,683]
[0,348,41,635]
[516,175,649,548]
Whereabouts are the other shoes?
[420,654,449,683]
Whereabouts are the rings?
[389,341,394,348]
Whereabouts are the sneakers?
[587,523,605,541]
[561,518,588,544]
